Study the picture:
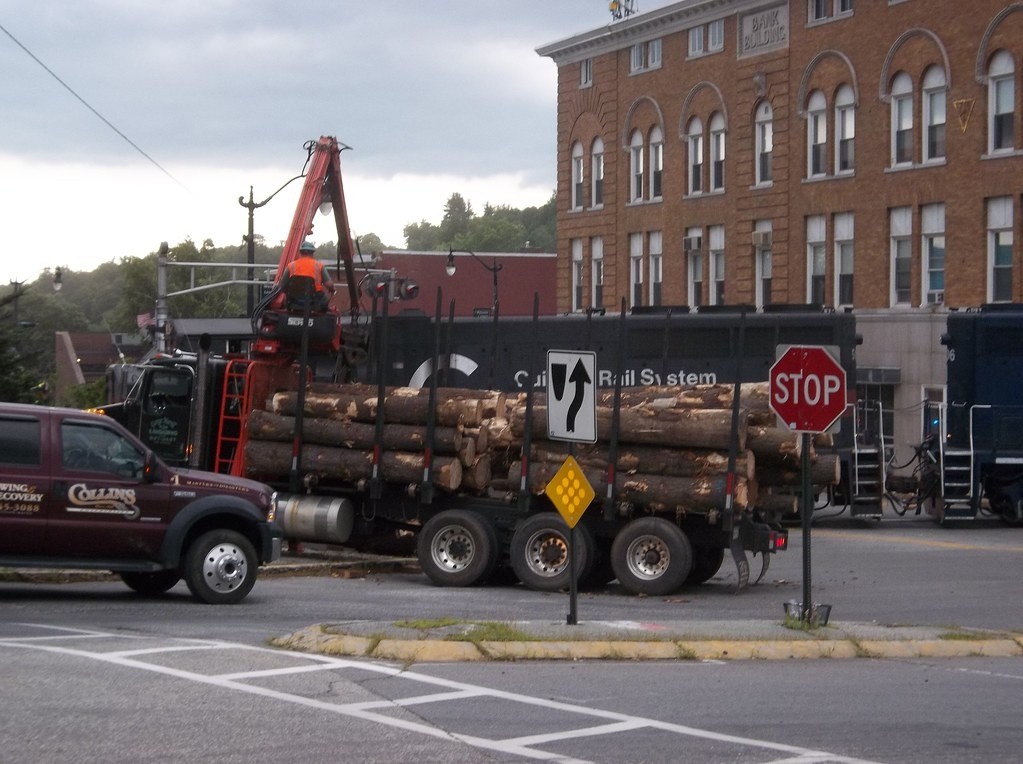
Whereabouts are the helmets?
[301,241,315,251]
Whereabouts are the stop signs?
[766,344,848,431]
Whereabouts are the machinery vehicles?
[215,133,367,480]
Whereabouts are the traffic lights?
[366,277,419,301]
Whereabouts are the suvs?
[0,403,281,602]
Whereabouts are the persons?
[278,242,338,313]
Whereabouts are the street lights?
[9,267,65,327]
[241,173,334,316]
[446,241,502,312]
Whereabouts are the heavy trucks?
[102,331,790,599]
